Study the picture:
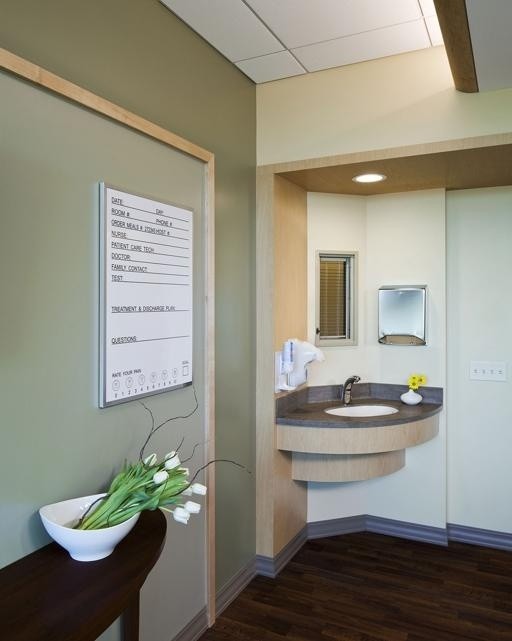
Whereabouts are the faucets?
[343,376,360,404]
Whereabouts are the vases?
[399,388,424,406]
[38,491,143,562]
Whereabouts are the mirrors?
[316,253,350,341]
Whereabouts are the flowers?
[71,450,209,525]
[407,372,426,388]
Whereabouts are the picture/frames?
[99,180,196,410]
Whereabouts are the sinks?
[323,404,399,417]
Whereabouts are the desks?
[0,487,172,641]
